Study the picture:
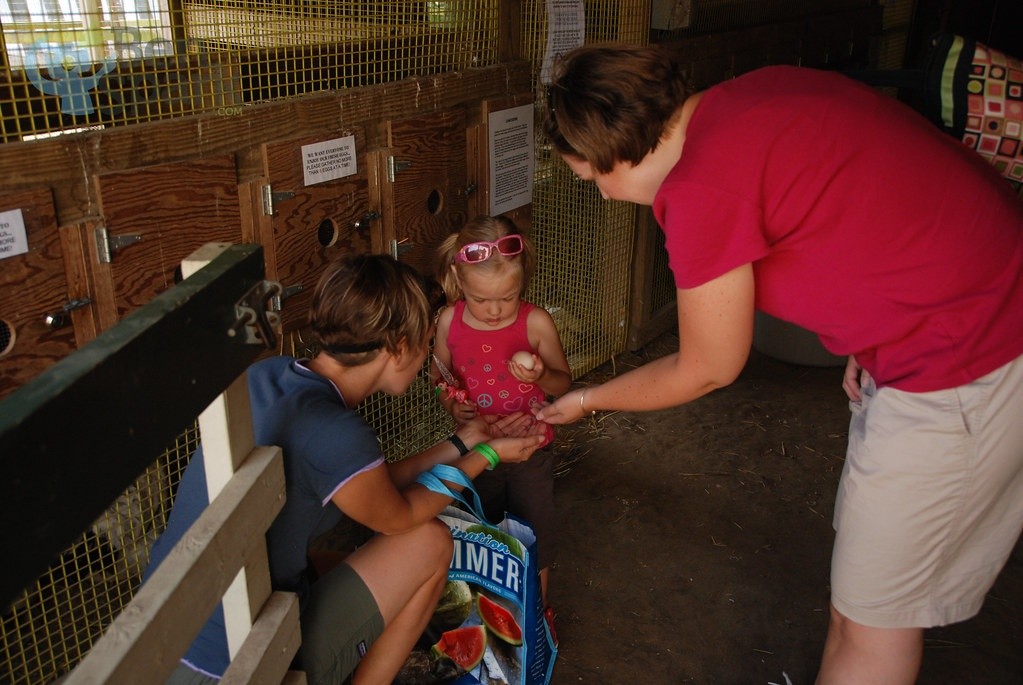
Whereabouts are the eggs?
[510,352,536,372]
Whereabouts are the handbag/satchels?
[395,465,559,685]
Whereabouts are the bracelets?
[447,434,469,457]
[581,386,595,417]
[472,443,501,470]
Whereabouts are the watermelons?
[426,582,523,672]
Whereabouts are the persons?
[430,213,574,649]
[137,250,551,684]
[528,40,1023,684]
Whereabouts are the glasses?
[456,235,524,263]
